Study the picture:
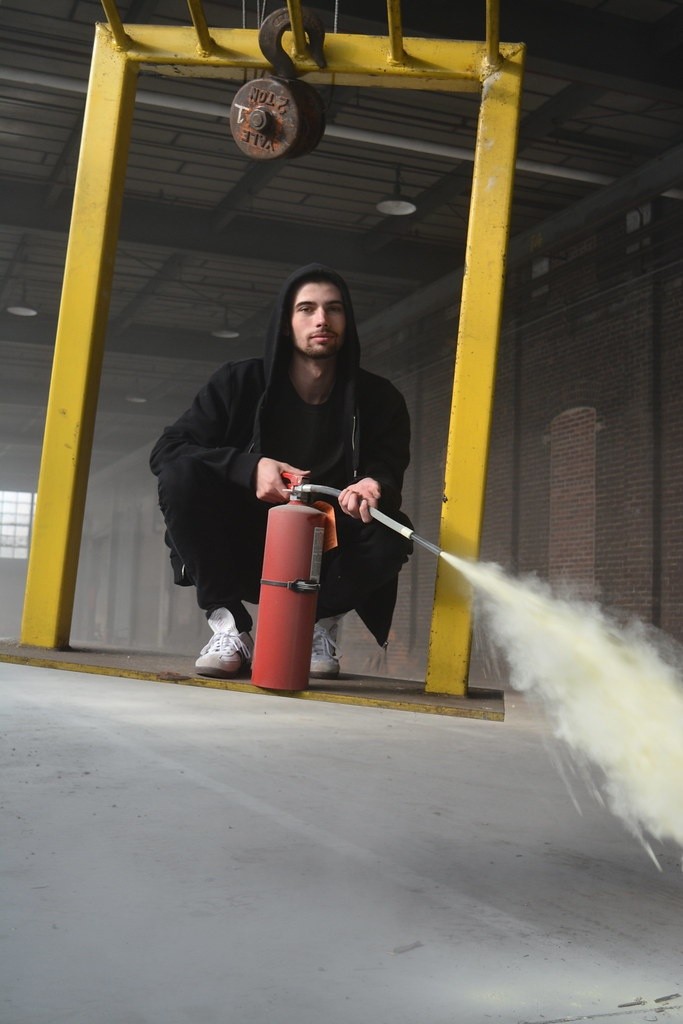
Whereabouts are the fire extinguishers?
[248,471,444,692]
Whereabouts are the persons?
[151,263,412,675]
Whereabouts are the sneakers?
[195,607,254,678]
[311,617,340,679]
